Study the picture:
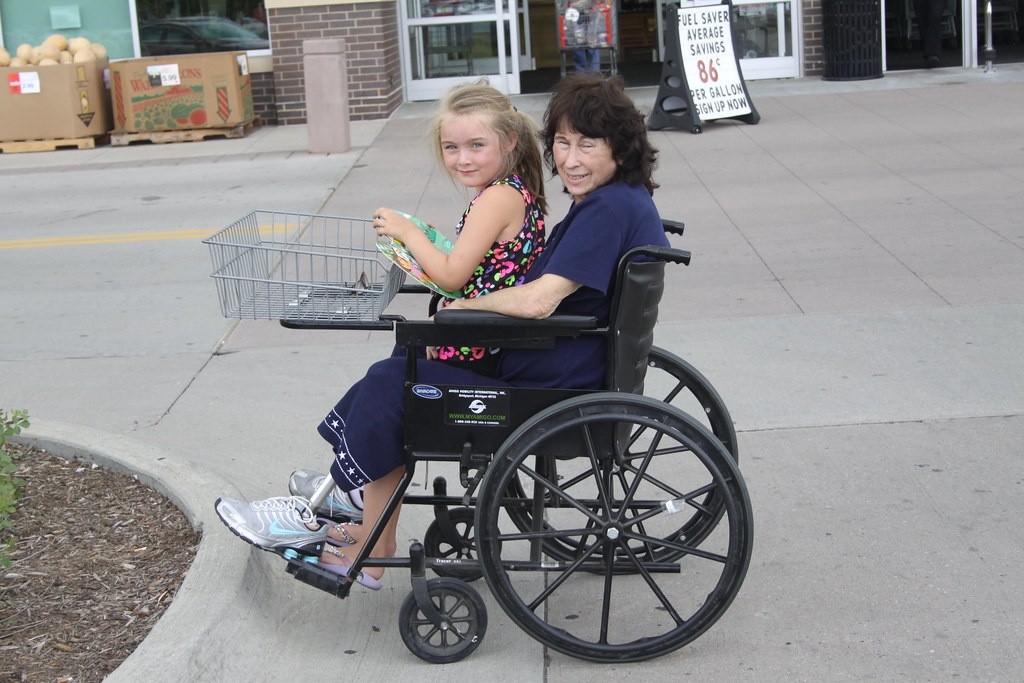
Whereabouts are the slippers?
[316,517,360,547]
[308,542,382,589]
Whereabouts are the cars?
[138,17,269,56]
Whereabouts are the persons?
[317,84,546,590]
[216,72,672,557]
[572,48,600,74]
[912,0,945,69]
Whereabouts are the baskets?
[202,209,407,322]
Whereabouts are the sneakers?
[214,497,330,562]
[289,469,364,525]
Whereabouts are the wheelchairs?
[202,210,756,664]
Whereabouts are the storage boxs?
[110,50,256,134]
[0,58,113,142]
